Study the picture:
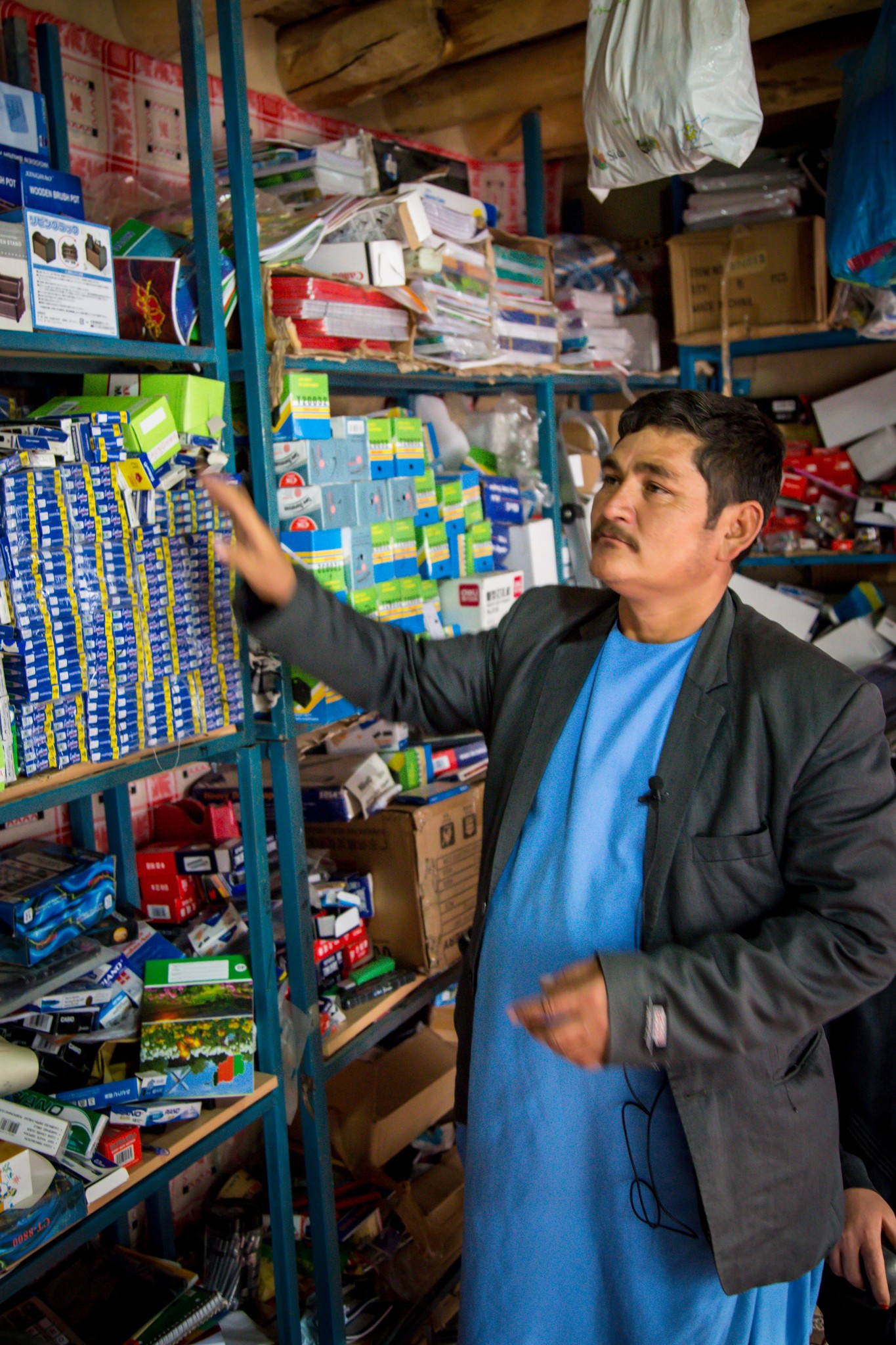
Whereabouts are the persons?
[821,663,896,1345]
[198,384,893,1344]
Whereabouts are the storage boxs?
[661,211,833,347]
[744,371,896,557]
[1,77,654,1248]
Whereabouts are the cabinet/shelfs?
[0,0,302,1345]
[682,334,895,699]
[212,0,687,1345]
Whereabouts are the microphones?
[648,776,666,807]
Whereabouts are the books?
[50,1244,232,1344]
